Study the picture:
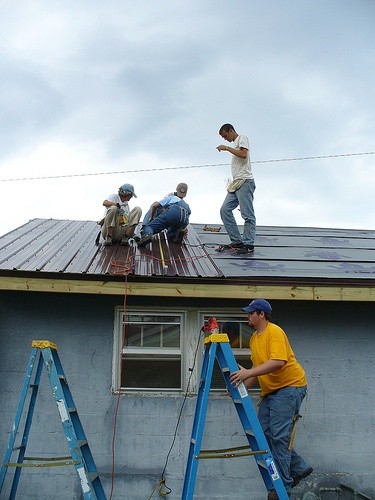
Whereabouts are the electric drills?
[199,317,219,337]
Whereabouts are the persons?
[216,123,256,255]
[137,183,191,247]
[226,299,313,500]
[97,184,142,245]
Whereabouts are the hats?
[120,184,137,198]
[176,183,188,198]
[241,299,273,314]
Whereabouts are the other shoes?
[120,234,129,245]
[137,234,152,247]
[269,489,280,500]
[291,467,313,488]
[104,234,112,245]
[173,230,184,243]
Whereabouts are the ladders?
[182,333,291,500]
[0,340,109,500]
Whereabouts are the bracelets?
[226,146,229,150]
[117,203,119,207]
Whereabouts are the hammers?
[288,414,302,450]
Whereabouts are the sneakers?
[225,243,244,248]
[238,245,255,254]
